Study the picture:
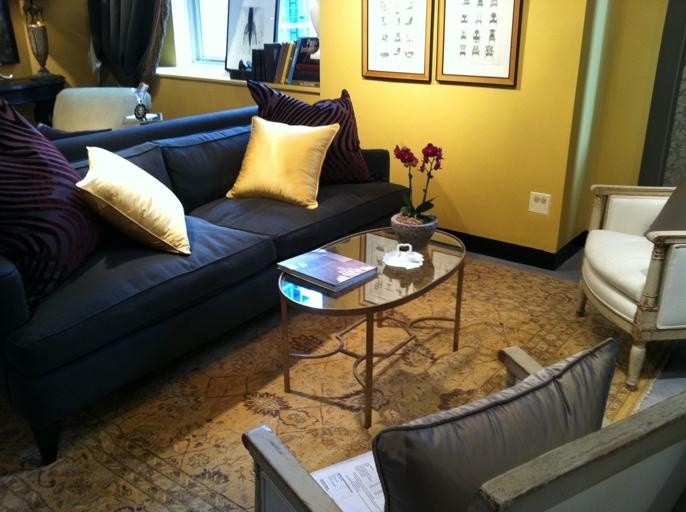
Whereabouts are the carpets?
[1,258,679,512]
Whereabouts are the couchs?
[52,85,153,134]
[577,183,686,391]
[0,100,411,466]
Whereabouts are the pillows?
[75,146,191,255]
[246,78,372,184]
[0,94,101,302]
[372,337,619,511]
[226,116,340,210]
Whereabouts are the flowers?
[393,143,447,214]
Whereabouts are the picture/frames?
[435,0,524,89]
[359,0,435,84]
[224,0,280,75]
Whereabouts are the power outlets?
[527,192,552,217]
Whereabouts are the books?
[276,247,378,294]
[251,39,319,86]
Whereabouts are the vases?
[391,216,440,250]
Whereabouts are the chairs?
[241,346,685,512]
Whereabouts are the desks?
[0,71,66,122]
